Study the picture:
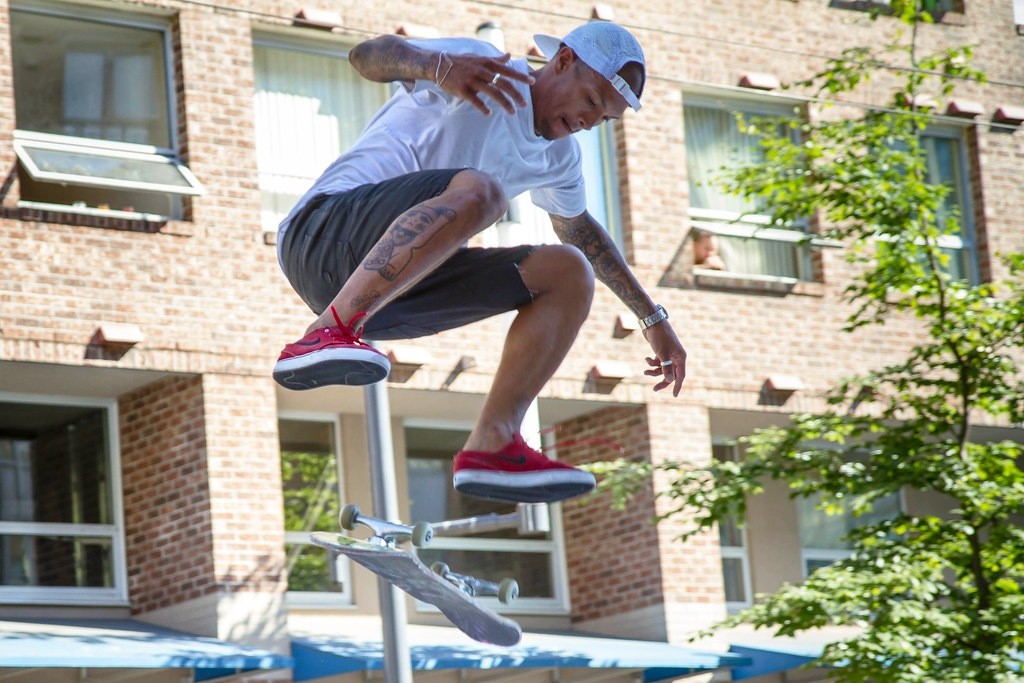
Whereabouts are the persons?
[269,22,690,504]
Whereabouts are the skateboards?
[312,503,524,648]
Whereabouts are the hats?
[532,20,646,112]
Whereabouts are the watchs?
[636,304,670,330]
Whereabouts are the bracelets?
[433,50,456,86]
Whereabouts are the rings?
[492,70,501,85]
[660,358,674,366]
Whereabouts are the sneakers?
[452,432,596,505]
[273,324,390,392]
